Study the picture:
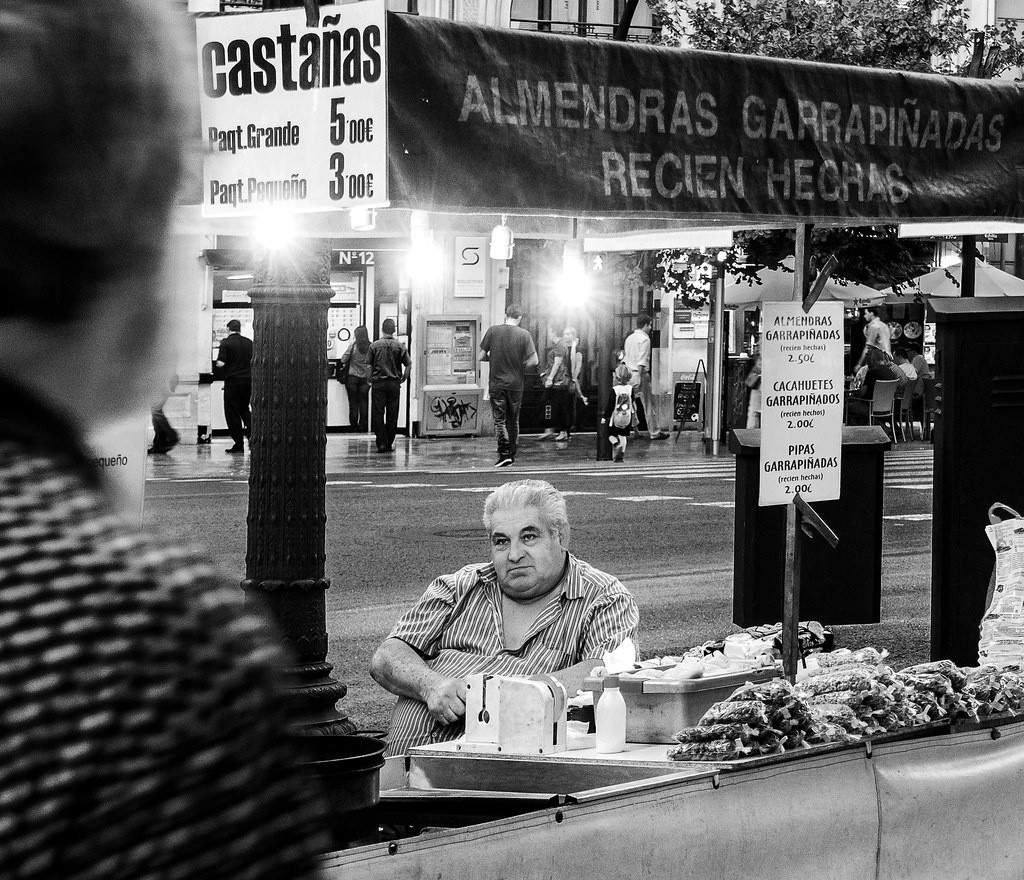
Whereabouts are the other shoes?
[495,453,516,467]
[149,447,173,454]
[635,434,645,438]
[554,430,572,440]
[225,443,244,453]
[613,445,625,462]
[538,428,555,440]
[651,432,670,440]
[378,441,392,452]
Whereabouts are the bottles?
[596,675,627,754]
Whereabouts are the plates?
[863,322,922,339]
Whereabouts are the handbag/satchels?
[538,361,571,391]
[977,502,1024,665]
[336,343,355,384]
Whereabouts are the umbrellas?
[880,257,1024,312]
[706,256,888,310]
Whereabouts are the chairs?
[895,377,920,443]
[921,377,935,441]
[845,376,902,444]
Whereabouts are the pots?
[272,729,389,818]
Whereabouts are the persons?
[365,318,411,453]
[744,338,761,429]
[539,320,588,440]
[147,406,177,454]
[0,0,328,880]
[217,321,254,453]
[478,304,539,466]
[370,479,641,783]
[341,326,371,432]
[624,313,670,440]
[851,308,931,439]
[600,365,635,463]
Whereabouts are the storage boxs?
[583,662,780,744]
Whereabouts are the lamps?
[489,215,515,260]
[408,211,434,243]
[348,208,378,231]
[561,217,587,271]
